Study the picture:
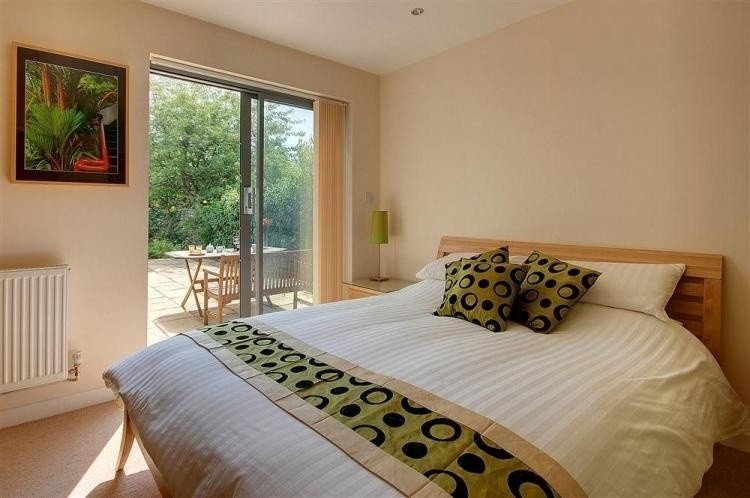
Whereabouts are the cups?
[187,242,225,253]
[252,244,257,252]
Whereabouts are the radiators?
[0,264,84,394]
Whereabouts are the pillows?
[415,244,688,335]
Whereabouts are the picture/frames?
[7,39,132,189]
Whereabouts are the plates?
[188,253,205,256]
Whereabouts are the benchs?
[202,248,314,325]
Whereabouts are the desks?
[164,243,288,319]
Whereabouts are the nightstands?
[337,276,417,300]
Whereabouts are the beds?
[113,235,725,498]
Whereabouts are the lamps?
[367,209,391,283]
